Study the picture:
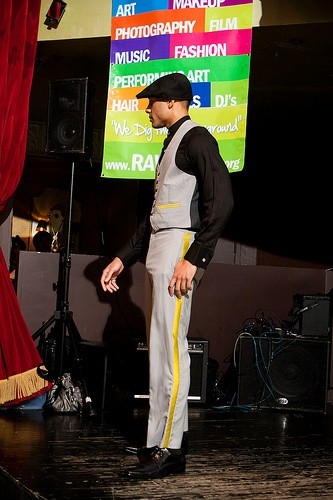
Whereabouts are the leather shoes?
[124,447,186,480]
[122,444,147,455]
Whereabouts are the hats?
[136,72,194,101]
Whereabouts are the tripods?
[28,158,98,418]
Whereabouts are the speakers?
[124,339,210,402]
[238,335,331,415]
[45,77,94,159]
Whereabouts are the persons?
[32,231,53,254]
[101,73,233,477]
